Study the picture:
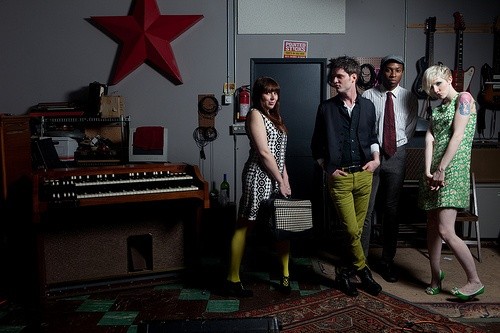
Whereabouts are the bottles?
[209,181,219,207]
[220,174,230,204]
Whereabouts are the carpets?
[0,263,485,333]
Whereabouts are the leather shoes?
[339,273,358,297]
[358,265,382,294]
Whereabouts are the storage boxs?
[101,96,126,118]
[263,199,314,233]
[470,148,500,183]
[40,137,78,161]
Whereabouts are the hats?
[381,55,405,65]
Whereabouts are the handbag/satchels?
[273,194,313,232]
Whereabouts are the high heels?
[426,270,445,295]
[450,285,485,299]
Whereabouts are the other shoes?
[382,259,399,282]
[222,280,254,298]
[280,275,292,299]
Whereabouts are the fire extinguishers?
[235,85,252,122]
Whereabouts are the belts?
[339,166,367,175]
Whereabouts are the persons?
[421,67,484,299]
[228,78,293,297]
[361,56,418,283]
[311,57,383,295]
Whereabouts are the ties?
[383,92,397,157]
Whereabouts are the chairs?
[455,171,483,263]
